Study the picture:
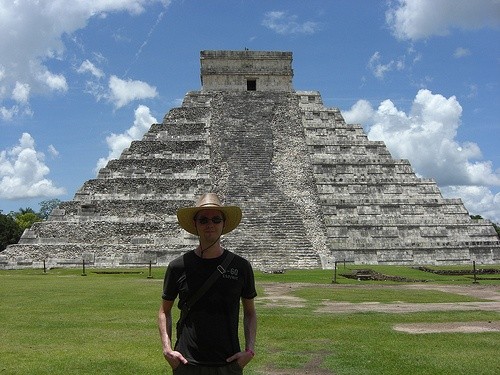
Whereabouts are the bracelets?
[245,349,255,356]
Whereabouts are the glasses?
[196,217,225,224]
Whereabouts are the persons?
[158,193,259,375]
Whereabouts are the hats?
[177,193,241,236]
